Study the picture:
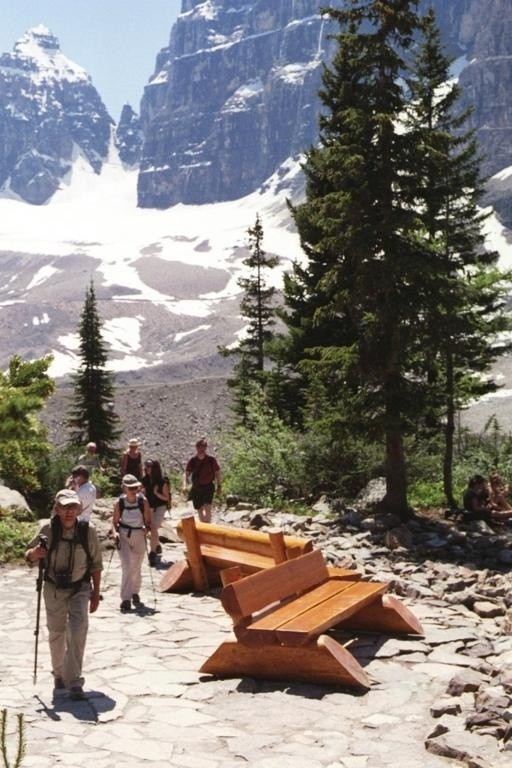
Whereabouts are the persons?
[141,458,171,560]
[120,438,146,480]
[184,438,222,523]
[113,473,152,612]
[24,488,102,701]
[77,441,107,477]
[64,464,98,527]
[463,473,511,523]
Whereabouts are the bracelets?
[217,484,221,488]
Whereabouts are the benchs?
[156,514,364,596]
[196,548,428,691]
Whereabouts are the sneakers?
[55,678,85,700]
[120,599,130,612]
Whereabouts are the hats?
[127,438,142,447]
[55,489,81,506]
[122,474,142,488]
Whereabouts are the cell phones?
[75,476,80,483]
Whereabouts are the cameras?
[57,571,67,587]
